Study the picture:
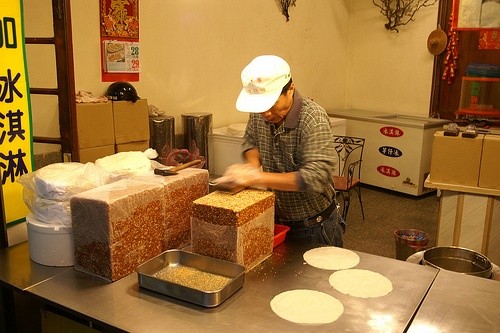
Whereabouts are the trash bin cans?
[394,229,429,261]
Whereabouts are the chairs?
[333,135,365,234]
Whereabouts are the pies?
[329,268,393,298]
[303,247,360,270]
[270,289,344,325]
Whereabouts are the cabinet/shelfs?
[460,77,500,116]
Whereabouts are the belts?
[276,200,337,228]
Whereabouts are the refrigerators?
[324,108,452,196]
[208,115,350,193]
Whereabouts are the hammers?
[154,159,202,177]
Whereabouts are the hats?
[235,55,291,113]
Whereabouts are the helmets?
[105,81,139,103]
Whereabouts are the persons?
[212,54,345,250]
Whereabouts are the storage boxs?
[431,130,500,189]
[136,249,245,307]
[274,224,290,248]
[76,99,150,163]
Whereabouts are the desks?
[0,174,500,333]
